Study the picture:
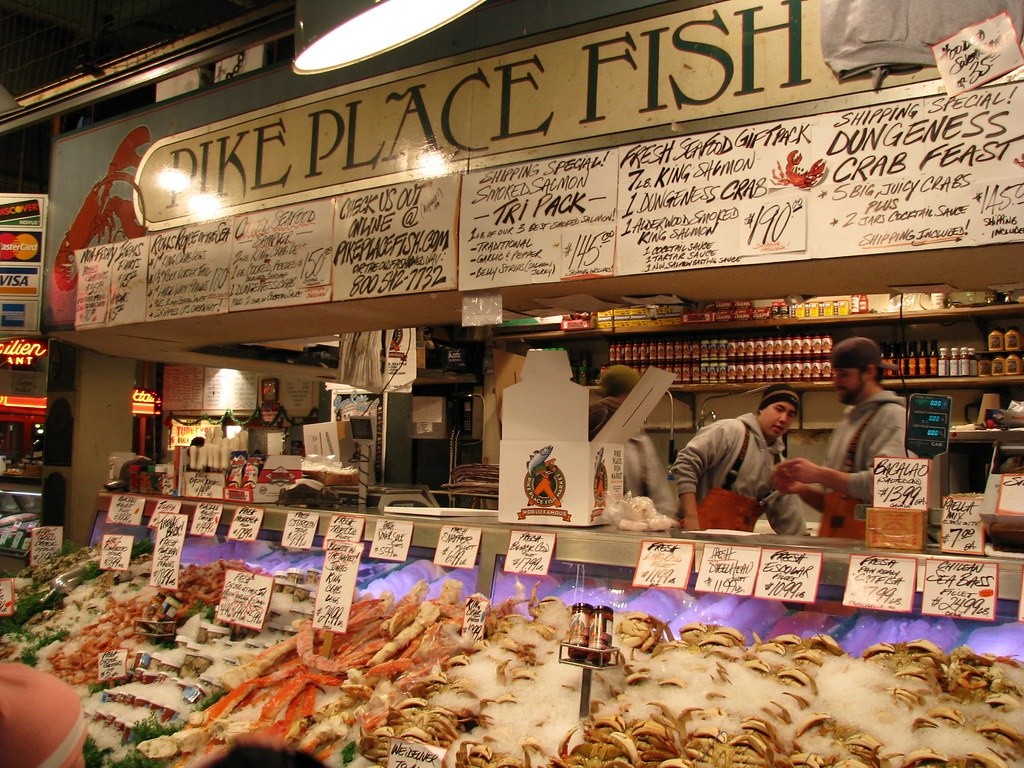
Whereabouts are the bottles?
[586,605,614,663]
[568,602,594,661]
[876,325,1024,378]
[851,294,868,314]
[129,463,174,496]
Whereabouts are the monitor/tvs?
[349,416,376,443]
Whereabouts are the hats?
[759,383,799,415]
[0,661,88,768]
[829,336,901,371]
[601,365,638,396]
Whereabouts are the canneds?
[568,602,614,663]
[84,651,207,750]
[138,592,182,634]
[771,302,789,319]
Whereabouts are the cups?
[133,653,151,670]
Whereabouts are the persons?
[587,366,680,527]
[671,384,811,536]
[767,338,918,540]
[0,662,89,768]
